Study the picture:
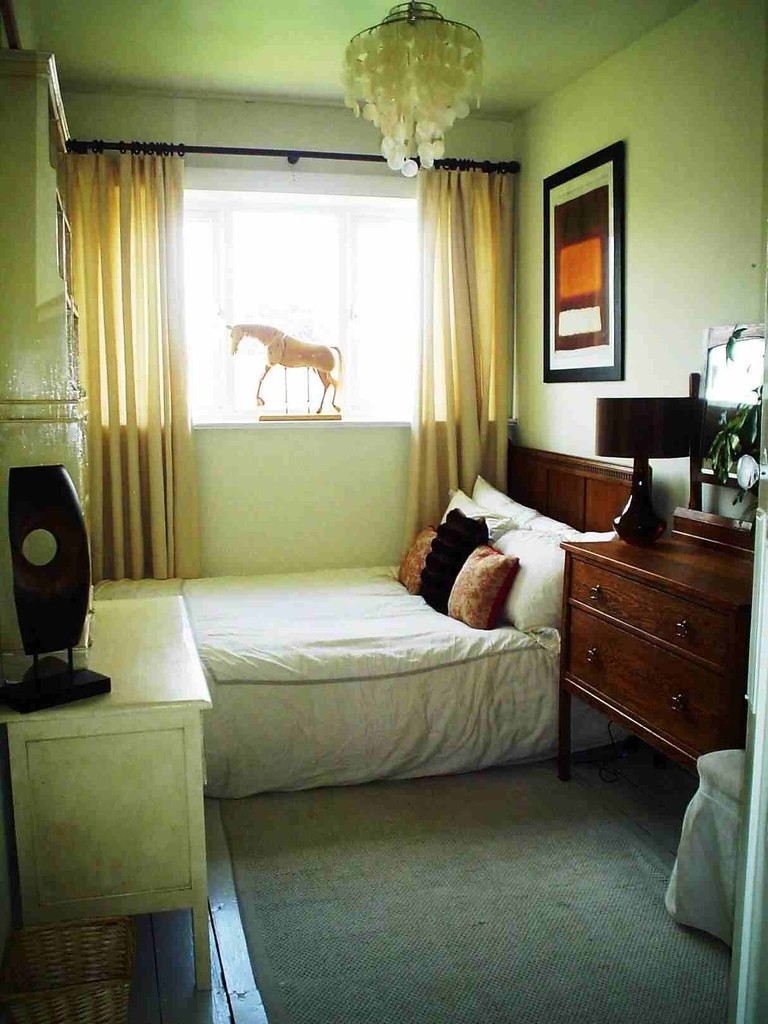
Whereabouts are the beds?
[93,420,649,802]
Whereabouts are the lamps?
[340,0,483,178]
[594,396,706,548]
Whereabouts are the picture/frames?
[542,138,625,384]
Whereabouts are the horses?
[226,324,344,414]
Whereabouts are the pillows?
[472,475,536,525]
[439,489,517,543]
[418,510,487,615]
[447,545,518,629]
[492,528,579,632]
[398,526,437,596]
[521,515,619,543]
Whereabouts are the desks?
[0,594,213,1003]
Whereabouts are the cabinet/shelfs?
[560,507,754,781]
